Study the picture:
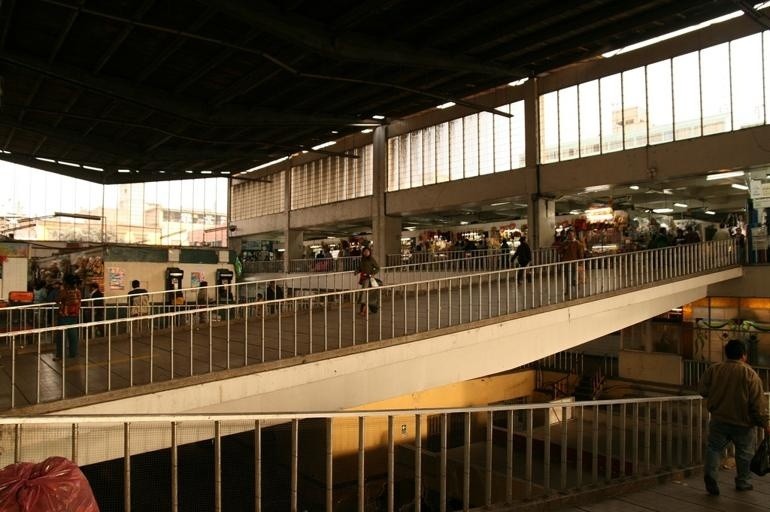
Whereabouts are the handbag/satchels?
[749,435,770,476]
[528,271,535,283]
[369,276,383,287]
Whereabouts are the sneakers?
[704,474,720,496]
[735,479,753,490]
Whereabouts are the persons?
[699,338,770,496]
[555,226,587,298]
[197,280,209,327]
[52,272,86,363]
[254,292,266,318]
[48,281,63,325]
[33,278,48,329]
[354,246,382,318]
[125,278,149,332]
[267,281,284,317]
[89,281,106,339]
[508,235,535,289]
